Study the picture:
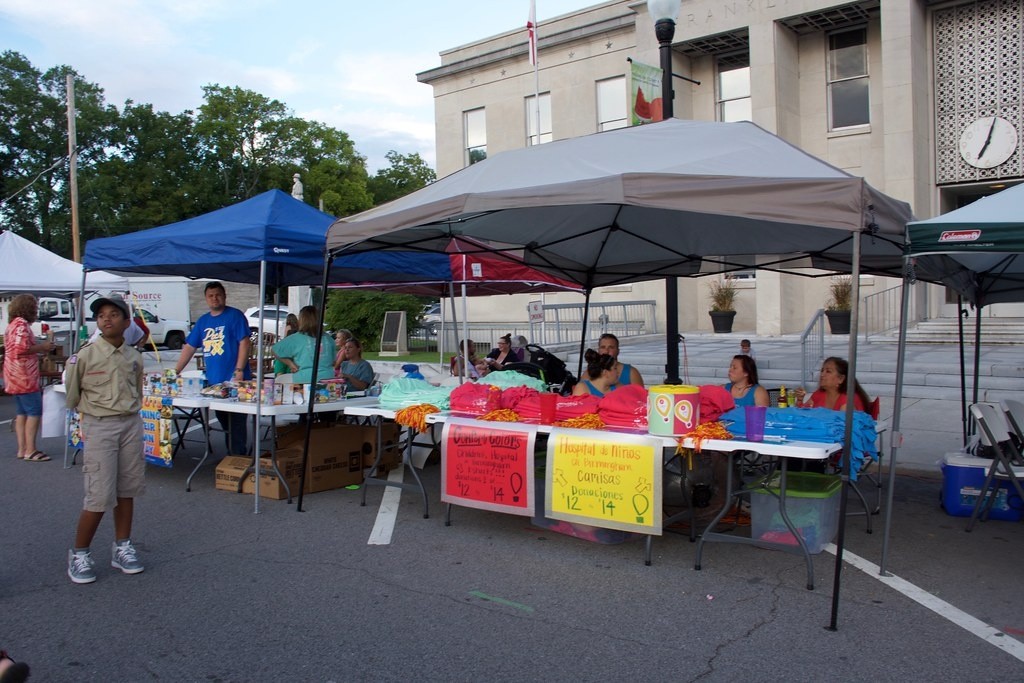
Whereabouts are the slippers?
[17,450,50,461]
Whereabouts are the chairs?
[964,403,1024,533]
[734,389,885,516]
[1001,400,1024,448]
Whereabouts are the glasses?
[346,339,356,342]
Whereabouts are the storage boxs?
[749,470,842,555]
[940,452,1024,522]
[282,383,294,404]
[273,383,282,404]
[215,423,399,500]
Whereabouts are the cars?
[415,302,441,336]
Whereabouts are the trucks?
[0,275,192,352]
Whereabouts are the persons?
[784,356,868,487]
[166,276,372,463]
[693,353,766,514]
[65,293,146,585]
[570,348,624,400]
[2,293,57,463]
[735,339,760,365]
[453,333,519,386]
[580,335,646,396]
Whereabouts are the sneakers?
[111,539,144,574]
[66,549,97,585]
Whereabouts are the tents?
[295,116,918,633]
[306,227,594,413]
[0,230,133,364]
[871,179,1024,581]
[69,187,456,517]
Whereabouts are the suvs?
[243,304,335,345]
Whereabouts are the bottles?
[55,361,65,372]
[42,320,51,335]
[777,385,795,408]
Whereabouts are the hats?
[90,298,131,318]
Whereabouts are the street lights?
[649,0,685,385]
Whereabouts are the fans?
[663,450,728,525]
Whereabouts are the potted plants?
[825,275,852,334]
[704,274,741,332]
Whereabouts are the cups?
[293,392,303,405]
[47,329,53,341]
[304,383,311,402]
[538,393,558,423]
[744,406,767,441]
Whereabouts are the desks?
[209,396,381,505]
[346,404,886,591]
[42,384,227,493]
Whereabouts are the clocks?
[959,116,1018,169]
